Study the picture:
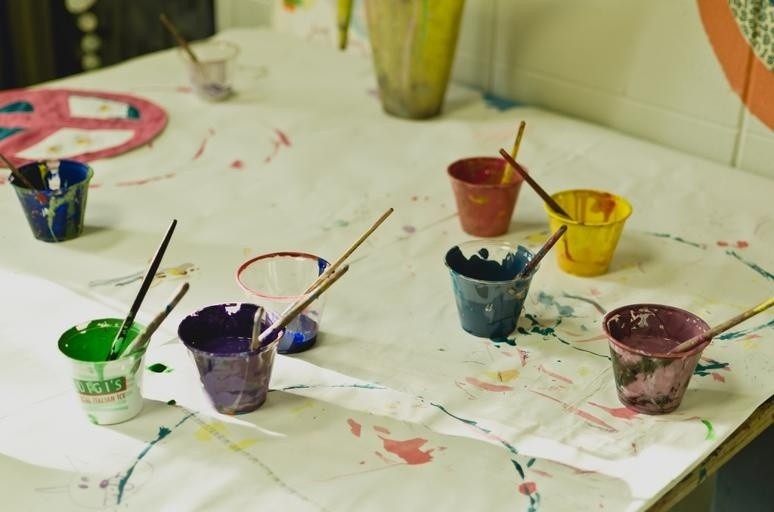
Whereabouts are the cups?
[56,317,152,428]
[176,300,287,418]
[602,302,714,417]
[541,187,634,279]
[441,237,541,340]
[177,38,240,104]
[444,156,529,240]
[236,251,335,357]
[7,158,95,245]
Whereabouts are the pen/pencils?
[248,207,396,350]
[159,12,200,62]
[499,121,572,281]
[107,220,189,361]
[0,153,33,188]
[667,296,774,354]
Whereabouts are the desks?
[0,22,774,512]
